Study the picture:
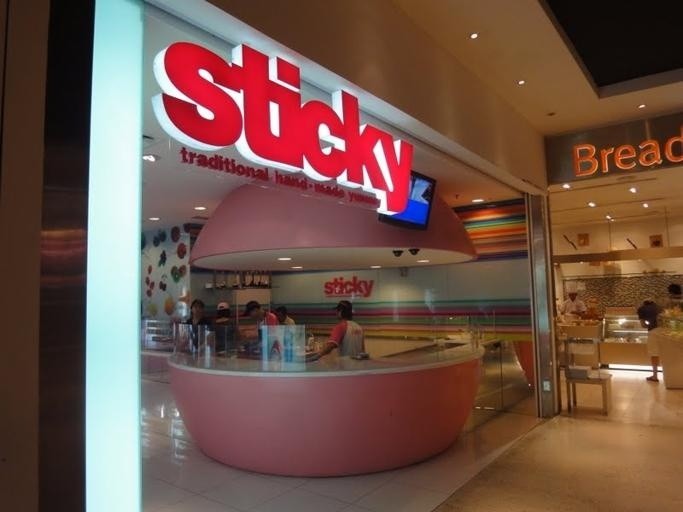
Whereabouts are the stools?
[566,374,611,417]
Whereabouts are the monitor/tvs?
[378,168,437,231]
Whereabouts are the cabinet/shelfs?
[599,307,661,366]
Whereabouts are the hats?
[332,301,352,310]
[216,301,261,316]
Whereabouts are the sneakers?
[646,376,659,382]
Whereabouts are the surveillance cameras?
[408,249,420,255]
[392,250,404,257]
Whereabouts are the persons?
[558,292,586,366]
[666,283,682,314]
[636,297,669,382]
[317,300,366,358]
[273,306,296,326]
[242,300,278,354]
[184,298,213,349]
[207,301,241,354]
